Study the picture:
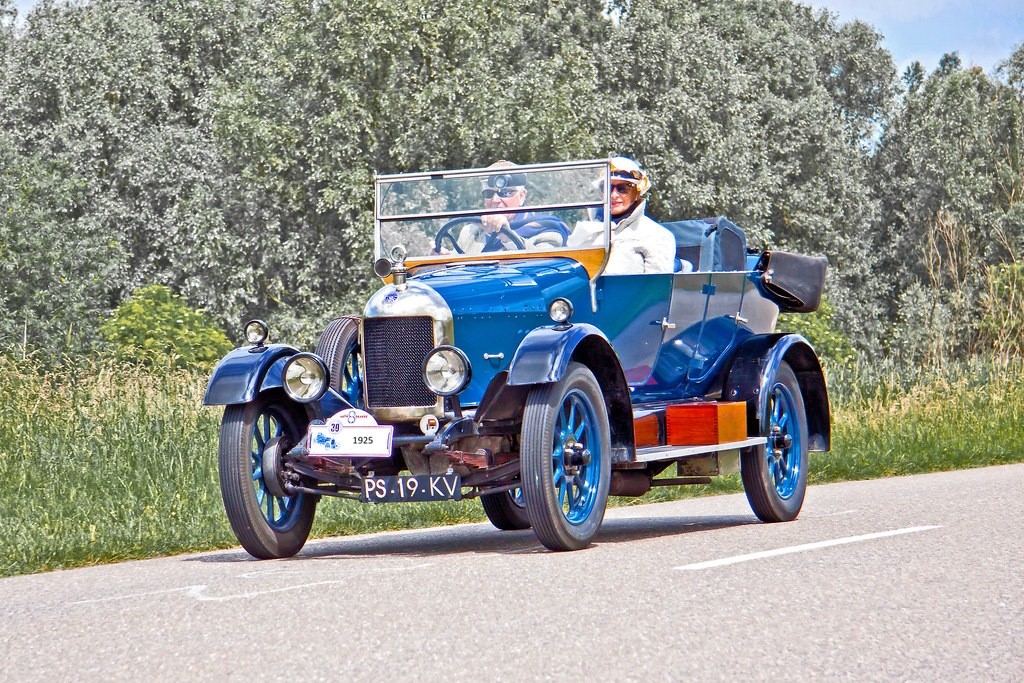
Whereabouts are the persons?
[566,156,676,274]
[424,160,571,254]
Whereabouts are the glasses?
[481,188,523,198]
[600,182,636,193]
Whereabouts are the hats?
[479,160,526,182]
[591,158,651,196]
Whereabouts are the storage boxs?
[666,400,747,446]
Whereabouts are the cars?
[201,161,832,559]
